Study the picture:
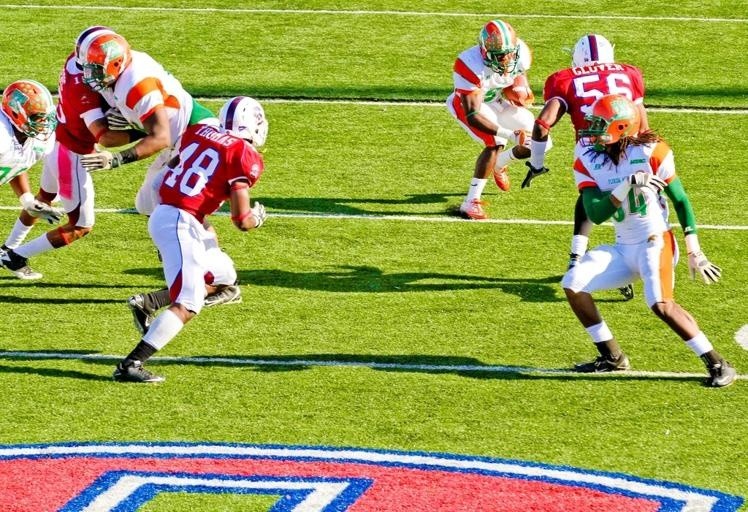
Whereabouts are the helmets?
[84,32,134,92]
[572,33,615,71]
[1,78,59,143]
[576,93,642,154]
[478,18,523,73]
[218,96,270,149]
[73,24,113,69]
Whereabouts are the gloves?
[628,171,668,195]
[510,129,534,148]
[106,109,130,127]
[18,192,67,225]
[686,250,725,286]
[521,160,550,189]
[250,200,268,229]
[80,142,124,174]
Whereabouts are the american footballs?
[503,75,529,106]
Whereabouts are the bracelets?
[495,126,513,139]
[609,178,631,203]
[683,232,700,254]
[528,139,547,161]
[119,147,139,164]
[126,128,142,143]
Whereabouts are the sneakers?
[0,249,44,280]
[203,280,243,308]
[127,293,152,335]
[112,359,166,384]
[458,198,491,220]
[493,166,510,192]
[573,351,631,374]
[706,359,737,389]
[618,284,634,301]
[567,253,581,271]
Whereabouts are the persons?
[557,93,738,388]
[0,25,151,281]
[0,77,67,226]
[75,26,245,306]
[442,17,555,223]
[111,93,270,384]
[518,29,653,301]
